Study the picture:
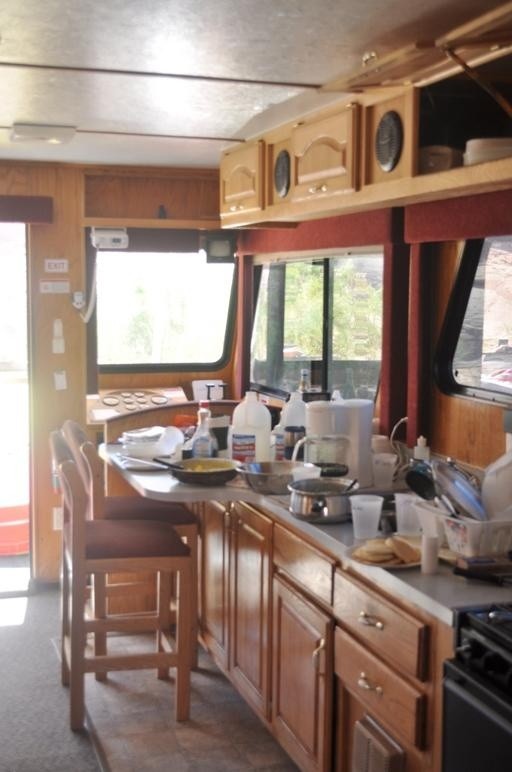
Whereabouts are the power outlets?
[413,435,430,460]
[52,509,69,530]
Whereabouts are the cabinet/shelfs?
[220,0,512,231]
[229,500,269,726]
[197,497,227,674]
[330,565,454,772]
[271,535,334,772]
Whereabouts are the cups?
[349,494,385,540]
[371,451,398,491]
[393,492,426,537]
[289,464,322,482]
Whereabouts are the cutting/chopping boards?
[395,532,512,572]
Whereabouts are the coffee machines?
[304,399,375,489]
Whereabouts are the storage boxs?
[87,388,289,634]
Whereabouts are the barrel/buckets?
[280,392,305,426]
[232,391,274,463]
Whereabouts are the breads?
[386,535,417,563]
[361,539,393,553]
[354,547,394,562]
[374,558,402,564]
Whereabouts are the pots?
[286,475,361,525]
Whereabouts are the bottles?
[284,426,306,461]
[299,369,308,392]
[192,409,212,459]
[414,436,430,461]
[199,401,218,458]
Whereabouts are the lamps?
[9,121,77,147]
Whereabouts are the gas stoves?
[452,604,512,706]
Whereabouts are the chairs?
[79,441,199,683]
[60,462,192,731]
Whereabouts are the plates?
[349,551,425,570]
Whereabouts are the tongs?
[434,492,459,522]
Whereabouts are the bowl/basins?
[169,457,241,485]
[465,139,511,149]
[235,460,320,495]
[462,150,510,166]
